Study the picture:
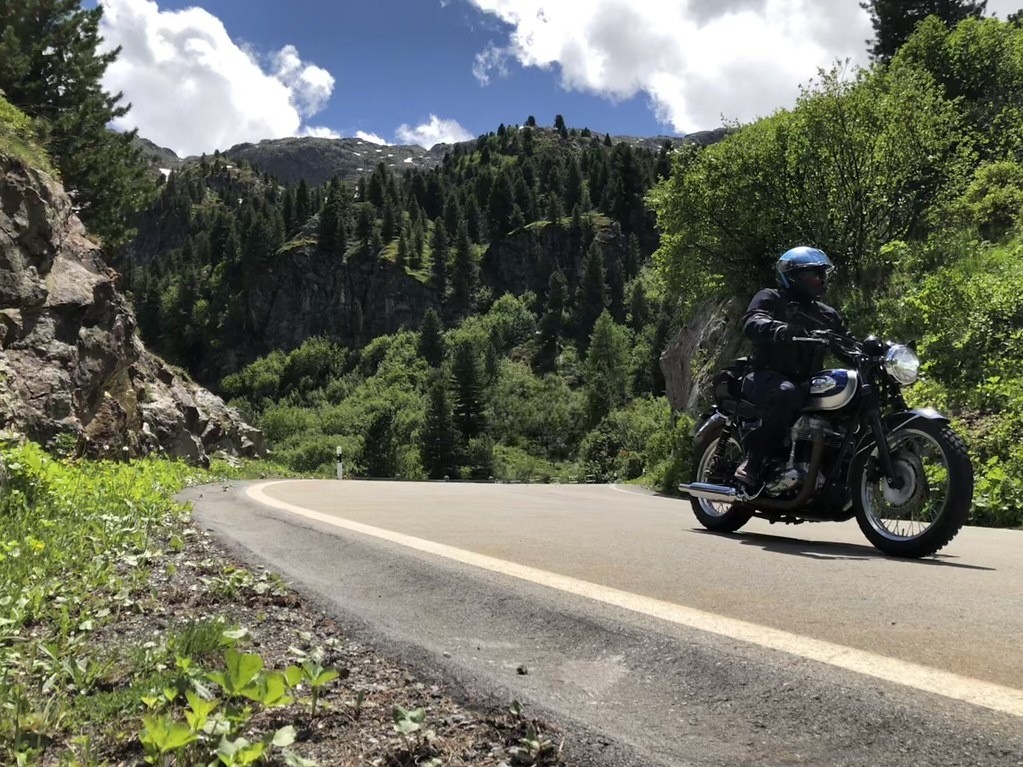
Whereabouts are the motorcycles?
[678,301,974,559]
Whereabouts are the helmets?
[776,247,832,300]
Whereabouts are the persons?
[734,246,861,491]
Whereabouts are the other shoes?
[734,448,768,487]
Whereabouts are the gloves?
[810,329,831,349]
